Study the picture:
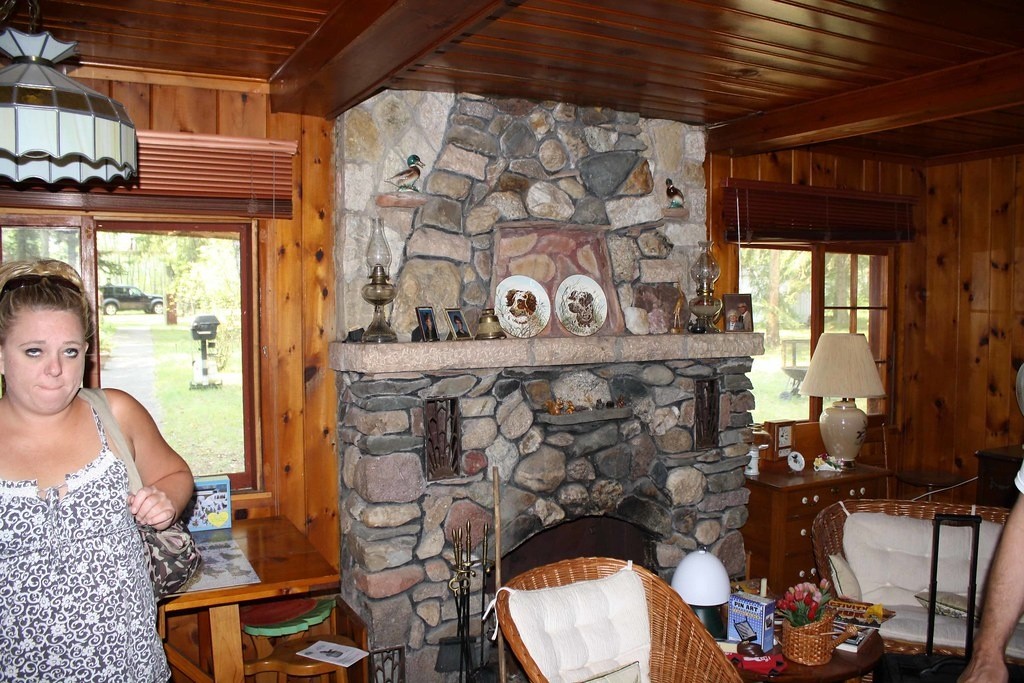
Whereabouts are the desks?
[157,514,341,683]
[738,632,883,683]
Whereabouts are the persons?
[423,312,438,340]
[0,259,195,683]
[453,315,469,338]
[727,301,752,332]
[956,458,1024,683]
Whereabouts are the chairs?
[495,558,745,683]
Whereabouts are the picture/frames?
[722,293,754,333]
[442,307,473,341]
[415,307,440,342]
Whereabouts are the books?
[296,640,369,667]
[826,595,897,653]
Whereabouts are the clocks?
[761,419,795,461]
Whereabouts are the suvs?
[99,285,163,315]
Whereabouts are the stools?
[245,634,359,683]
[895,469,966,501]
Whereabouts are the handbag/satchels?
[78,389,201,598]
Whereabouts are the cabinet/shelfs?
[737,460,895,597]
[974,445,1023,508]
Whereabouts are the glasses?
[1,275,82,298]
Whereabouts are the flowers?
[776,577,833,626]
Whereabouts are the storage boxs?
[727,591,775,652]
[180,474,232,533]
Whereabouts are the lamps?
[689,241,722,333]
[672,550,732,639]
[0,0,140,193]
[361,216,399,341]
[797,332,888,470]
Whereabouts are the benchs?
[811,496,1024,668]
